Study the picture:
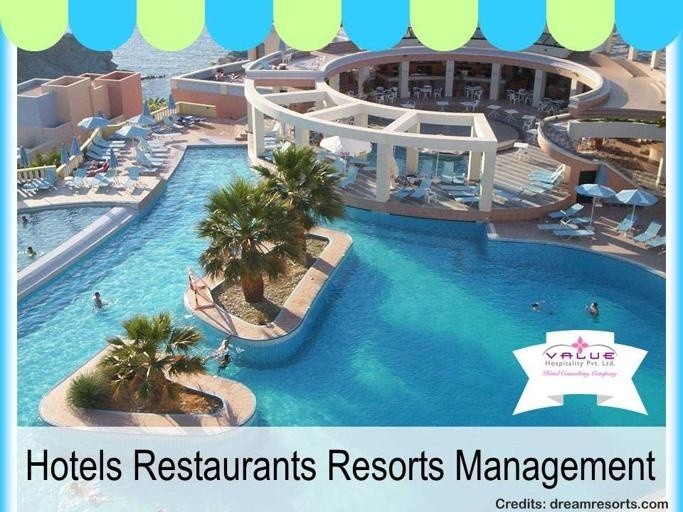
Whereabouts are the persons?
[219,354,230,368]
[214,70,219,81]
[26,247,37,258]
[85,157,109,179]
[589,302,599,316]
[220,71,224,81]
[92,292,103,308]
[88,159,99,172]
[218,339,229,353]
[532,302,541,312]
[20,216,30,226]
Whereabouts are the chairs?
[23,115,207,190]
[397,173,480,206]
[328,146,377,189]
[263,131,292,161]
[536,203,665,255]
[528,164,564,193]
[345,85,564,162]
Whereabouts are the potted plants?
[193,143,347,303]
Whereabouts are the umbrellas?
[413,131,468,178]
[18,145,30,169]
[615,188,658,222]
[60,142,70,165]
[278,39,288,64]
[77,116,112,142]
[320,134,373,174]
[168,93,177,117]
[109,148,119,174]
[142,100,152,119]
[97,110,108,135]
[114,125,151,152]
[69,136,80,157]
[124,114,157,131]
[573,183,616,226]
